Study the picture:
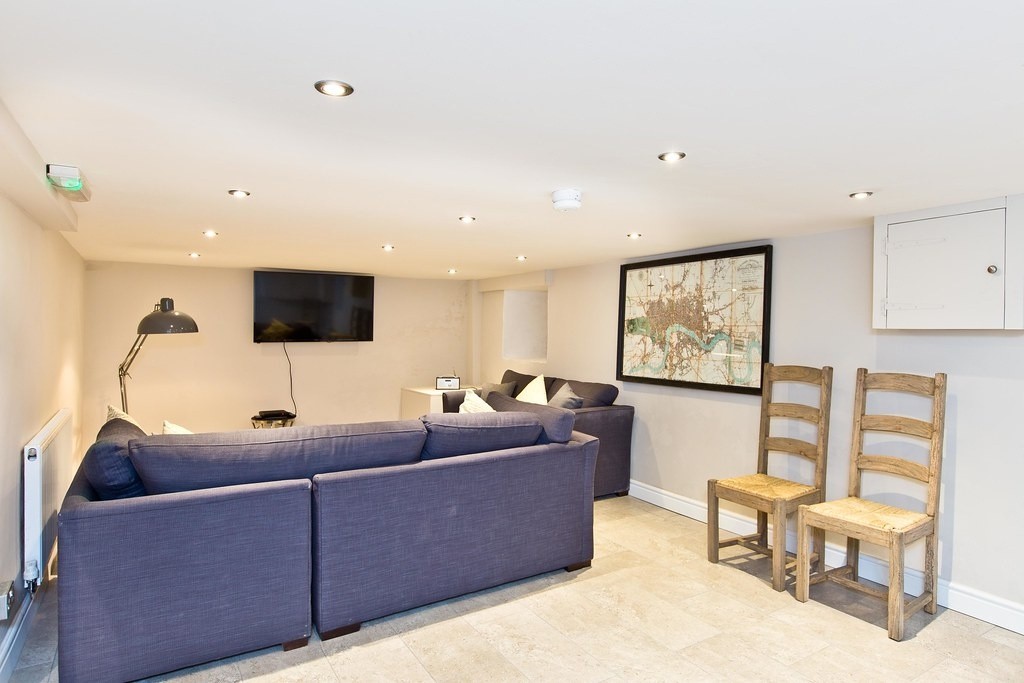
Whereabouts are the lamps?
[119,296,198,414]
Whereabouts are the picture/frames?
[613,245,771,398]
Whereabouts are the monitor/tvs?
[252,270,374,342]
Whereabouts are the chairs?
[797,368,947,641]
[706,362,834,592]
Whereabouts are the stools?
[251,414,297,429]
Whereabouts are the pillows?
[81,369,618,502]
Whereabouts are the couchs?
[57,430,598,683]
[443,369,635,497]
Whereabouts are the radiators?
[23,408,71,587]
